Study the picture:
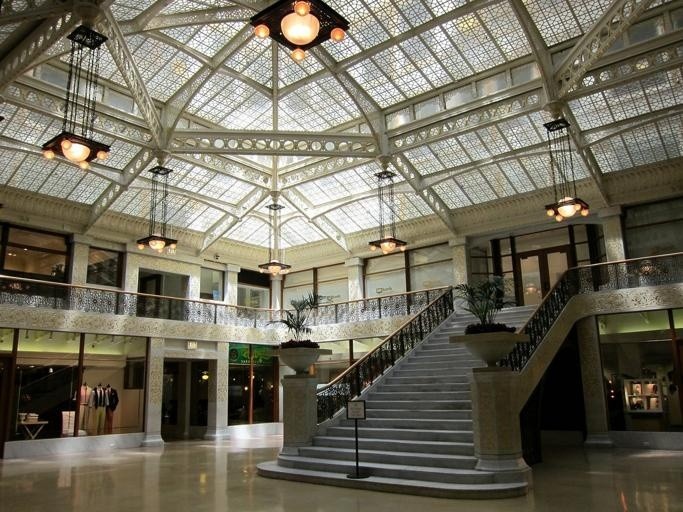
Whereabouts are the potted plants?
[442,274,540,367]
[263,291,340,373]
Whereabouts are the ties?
[98,389,103,407]
[107,389,110,400]
[84,389,87,404]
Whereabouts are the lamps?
[247,0,352,62]
[539,116,592,222]
[134,165,179,256]
[43,25,111,169]
[259,203,294,276]
[366,169,413,256]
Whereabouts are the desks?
[17,421,48,440]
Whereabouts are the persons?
[88,382,109,435]
[73,382,92,434]
[105,383,118,433]
